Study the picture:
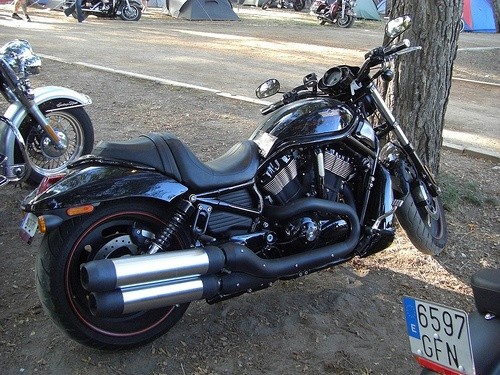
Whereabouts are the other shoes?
[11,13,22,20]
[27,18,31,22]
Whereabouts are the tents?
[460,0,499,33]
[161,0,241,21]
[310,0,380,20]
[377,0,393,16]
[243,0,277,7]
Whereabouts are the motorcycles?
[262,0,305,12]
[402,267,500,375]
[75,0,146,20]
[1,38,95,188]
[21,15,446,353]
[314,0,356,28]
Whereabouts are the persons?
[140,0,148,12]
[11,0,31,22]
